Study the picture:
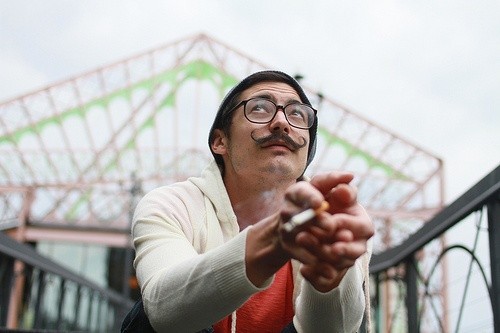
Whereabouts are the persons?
[119,71,375,333]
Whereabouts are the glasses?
[223,98,318,130]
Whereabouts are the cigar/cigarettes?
[282,200,331,232]
